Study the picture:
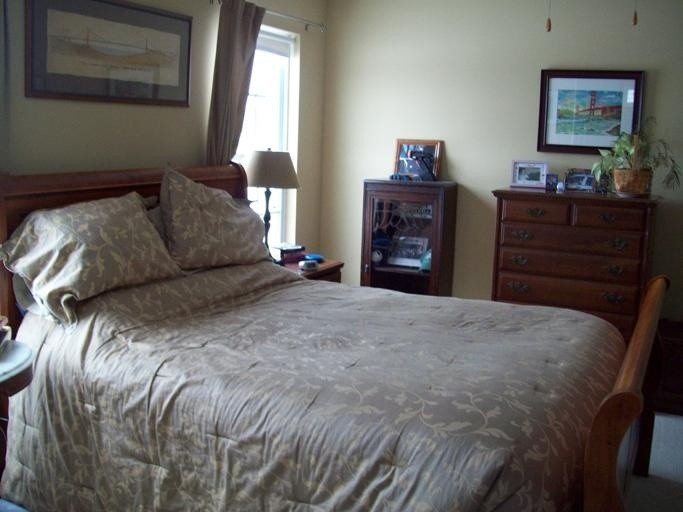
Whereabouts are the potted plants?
[592,123,681,198]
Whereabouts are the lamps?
[242,148,299,250]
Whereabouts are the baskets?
[613,135,651,193]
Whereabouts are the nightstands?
[268,255,344,282]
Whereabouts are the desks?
[0,339,34,479]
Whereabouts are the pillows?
[1,190,183,327]
[157,160,272,271]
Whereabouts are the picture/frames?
[509,160,546,188]
[536,68,648,155]
[24,0,191,108]
[393,139,441,180]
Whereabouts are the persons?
[518,168,529,181]
[398,245,422,259]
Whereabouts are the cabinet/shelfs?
[360,179,454,300]
[488,190,655,327]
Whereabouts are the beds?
[0,161,669,512]
